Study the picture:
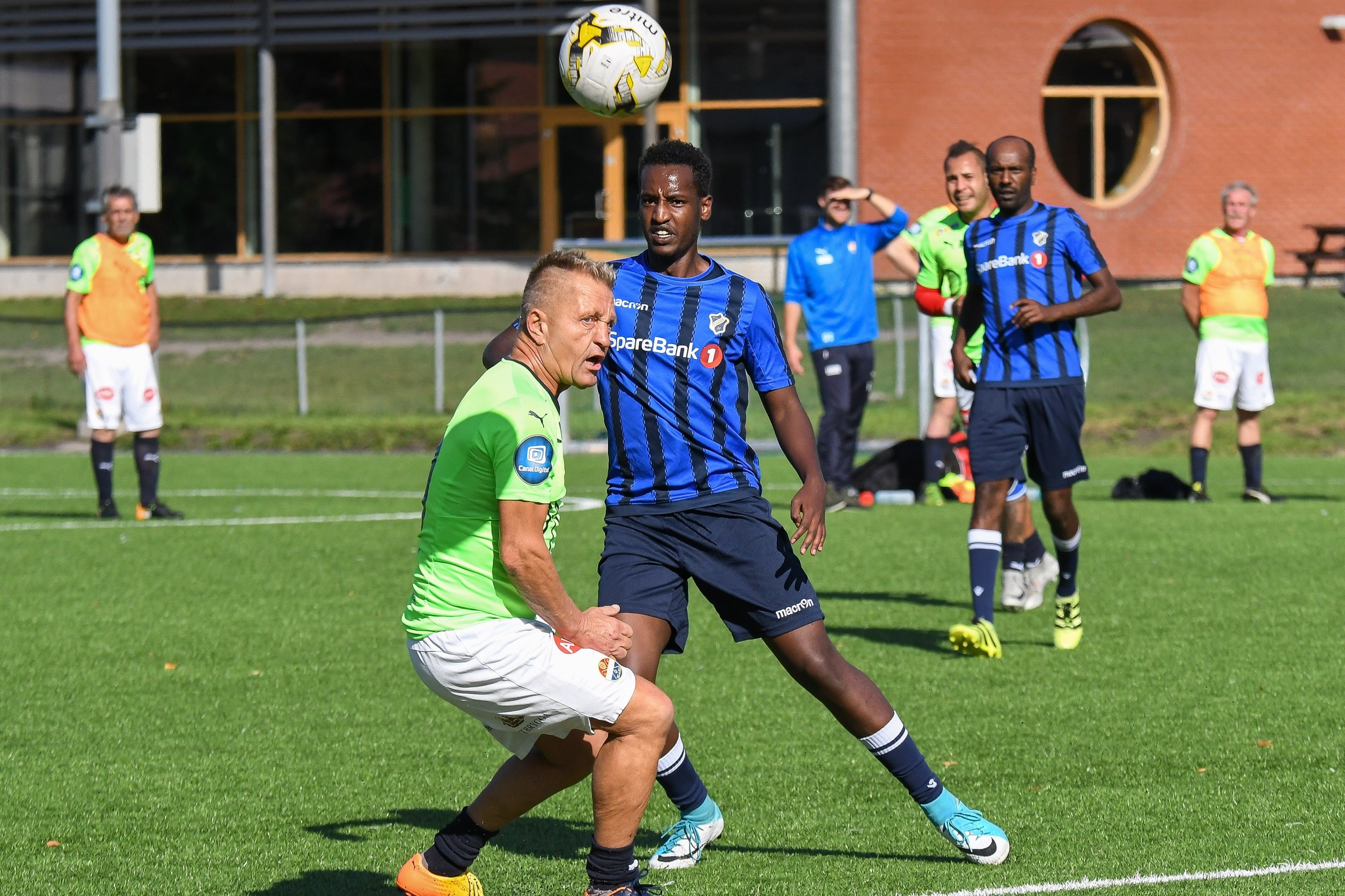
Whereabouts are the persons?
[63,186,185,521]
[482,137,1012,868]
[1182,180,1284,506]
[886,139,1061,613]
[782,176,909,514]
[390,248,675,896]
[946,134,1123,660]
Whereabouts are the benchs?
[1283,250,1345,289]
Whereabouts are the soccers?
[558,5,672,115]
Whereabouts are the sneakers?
[137,497,184,521]
[649,802,723,871]
[1054,584,1083,649]
[395,851,484,896]
[1017,549,1062,613]
[919,786,1010,866]
[1001,567,1024,612]
[95,497,122,520]
[585,867,662,896]
[946,618,1003,659]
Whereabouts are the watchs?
[865,188,874,200]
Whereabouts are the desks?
[1300,223,1345,286]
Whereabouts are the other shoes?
[1242,487,1285,502]
[836,485,860,507]
[924,485,943,506]
[1193,492,1210,502]
[823,484,845,513]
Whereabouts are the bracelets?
[511,318,520,329]
